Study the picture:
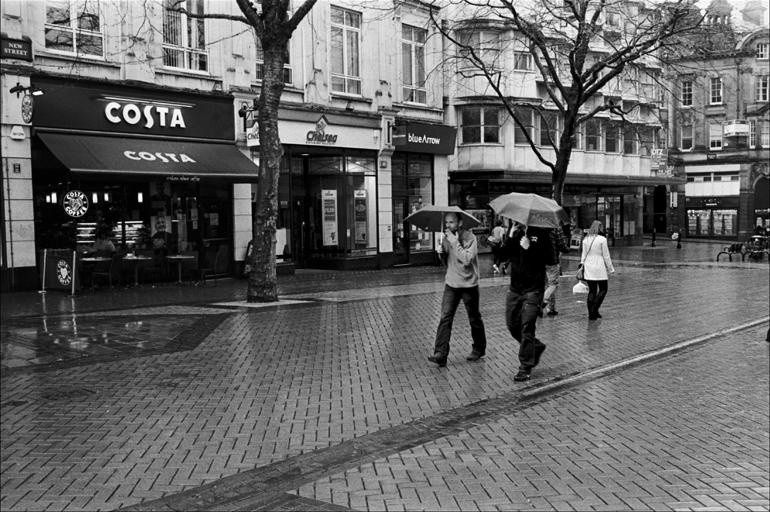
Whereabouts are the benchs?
[716,238,770,263]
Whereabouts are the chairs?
[73,237,232,290]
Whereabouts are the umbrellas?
[403,203,482,247]
[488,192,566,237]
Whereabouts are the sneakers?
[588,311,601,320]
[466,351,487,360]
[538,305,558,317]
[514,345,546,382]
[428,353,447,365]
[492,264,507,275]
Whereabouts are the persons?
[486,219,614,320]
[505,219,562,382]
[427,211,487,367]
[151,215,171,239]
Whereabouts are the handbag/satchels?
[576,265,583,280]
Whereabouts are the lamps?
[11,82,45,124]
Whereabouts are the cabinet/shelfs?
[72,216,147,251]
[393,191,428,248]
